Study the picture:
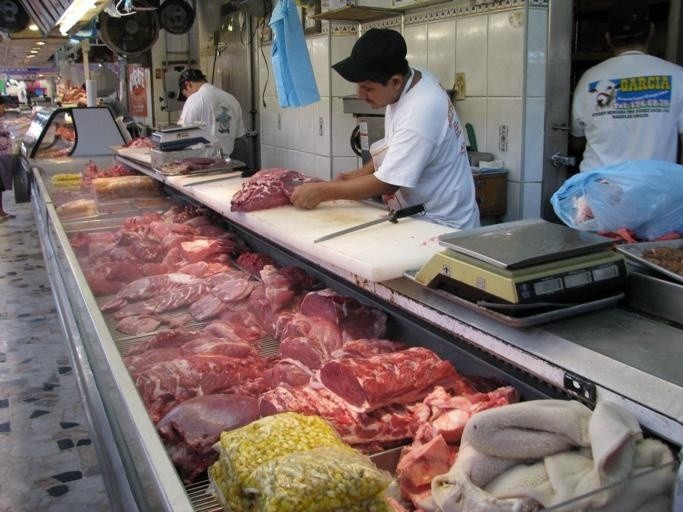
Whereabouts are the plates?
[148,155,251,175]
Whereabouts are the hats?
[176,69,204,101]
[331,28,408,82]
[604,3,650,35]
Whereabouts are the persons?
[77,97,139,139]
[0,96,16,224]
[291,29,482,231]
[176,67,245,155]
[570,0,683,168]
[80,83,87,95]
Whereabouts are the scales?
[150,124,212,152]
[415,218,627,318]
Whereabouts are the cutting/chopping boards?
[178,177,439,275]
